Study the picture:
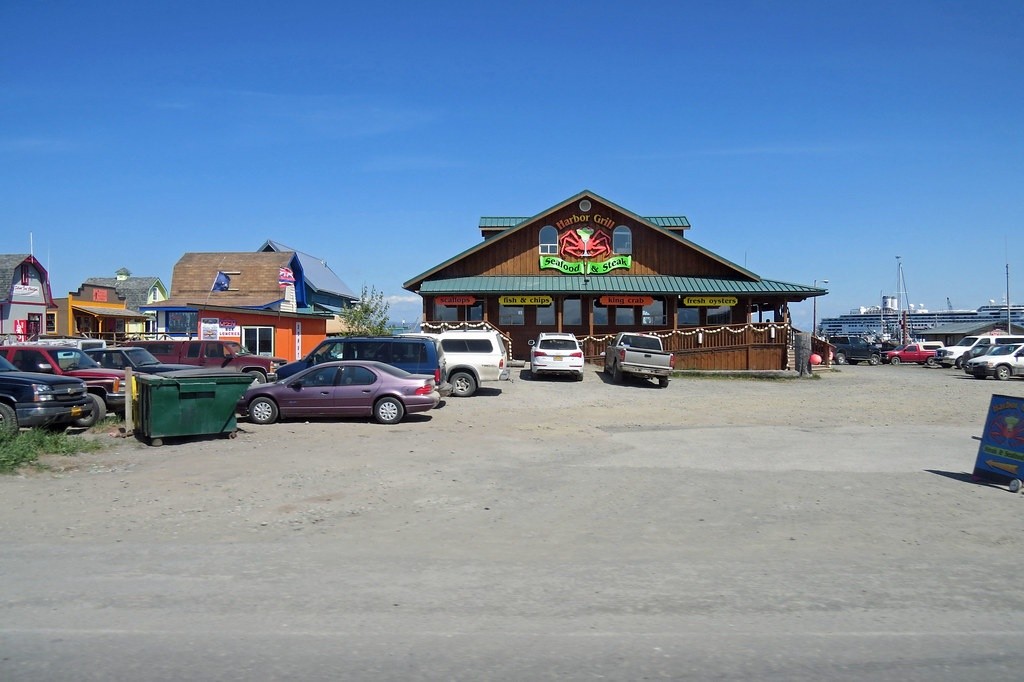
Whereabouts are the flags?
[278,267,296,288]
[210,271,230,291]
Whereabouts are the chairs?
[349,347,356,359]
[105,353,117,368]
[93,354,102,363]
[210,349,217,357]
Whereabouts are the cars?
[914,335,1024,381]
[0,333,288,441]
[234,360,442,424]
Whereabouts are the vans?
[272,333,453,400]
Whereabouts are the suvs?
[528,331,585,381]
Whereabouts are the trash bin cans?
[134,367,254,447]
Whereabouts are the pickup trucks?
[604,331,676,387]
[361,328,508,397]
[825,335,882,365]
[885,342,937,367]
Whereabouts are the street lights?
[813,279,829,353]
